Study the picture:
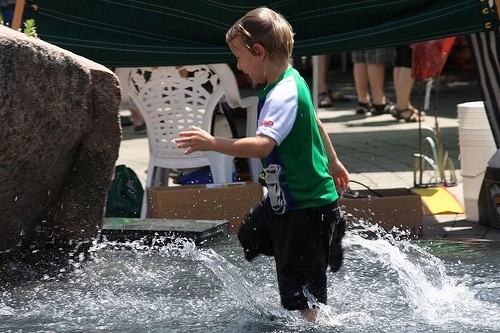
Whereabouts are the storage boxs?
[339,187,424,242]
[146,182,263,237]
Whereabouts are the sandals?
[329,88,343,101]
[370,102,392,115]
[318,92,333,107]
[356,102,371,114]
[391,106,425,122]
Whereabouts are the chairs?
[115,63,264,224]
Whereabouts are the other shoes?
[327,218,346,271]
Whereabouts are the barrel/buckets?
[456,100,497,223]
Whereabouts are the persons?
[352,43,424,122]
[318,55,342,107]
[175,7,350,320]
[113,66,187,131]
